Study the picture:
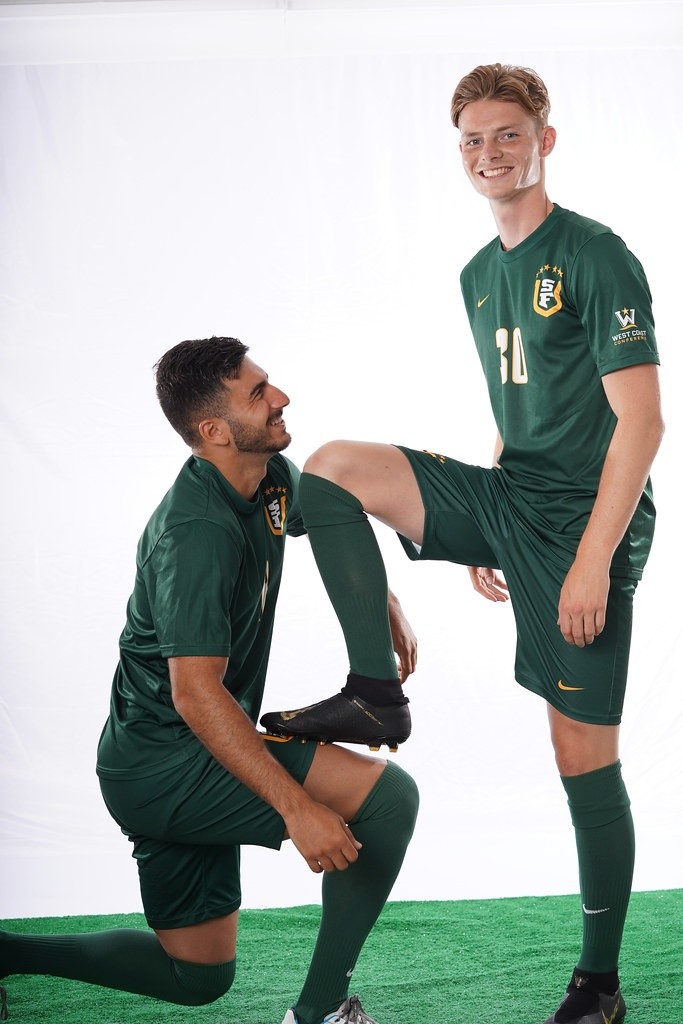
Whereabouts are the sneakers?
[259,692,412,752]
[543,974,627,1024]
[283,999,378,1024]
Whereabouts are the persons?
[257,61,666,1024]
[0,334,420,1024]
